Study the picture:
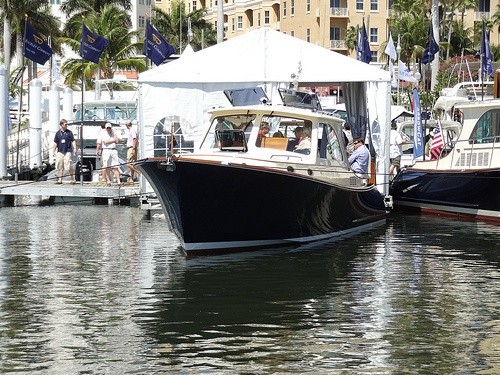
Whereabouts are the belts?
[104,148,117,150]
[127,146,133,150]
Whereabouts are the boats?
[134,85,388,258]
[386,80,500,225]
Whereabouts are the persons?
[389,123,406,177]
[125,121,140,182]
[215,116,235,142]
[53,119,77,183]
[293,120,312,150]
[258,122,283,142]
[329,122,353,146]
[347,137,369,178]
[96,122,121,185]
[424,127,434,143]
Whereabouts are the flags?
[357,24,372,63]
[23,22,52,64]
[80,24,109,65]
[398,60,416,82]
[389,62,398,88]
[385,35,397,61]
[479,26,494,79]
[422,25,440,64]
[142,21,174,66]
[431,121,443,162]
[413,87,423,160]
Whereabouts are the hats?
[105,122,112,129]
[293,127,304,133]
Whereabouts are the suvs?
[50,120,136,181]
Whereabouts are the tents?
[136,28,392,194]
